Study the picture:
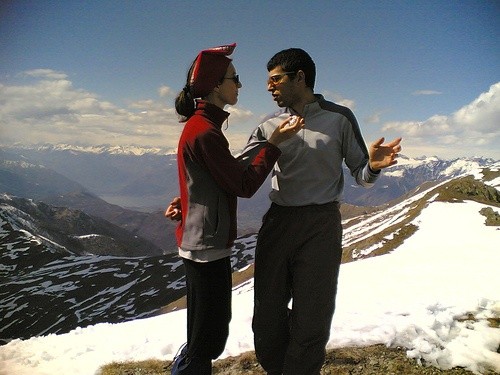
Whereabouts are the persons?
[170,42,306,375]
[165,47,404,375]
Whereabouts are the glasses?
[221,75,239,84]
[266,72,298,86]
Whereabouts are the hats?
[188,43,236,94]
